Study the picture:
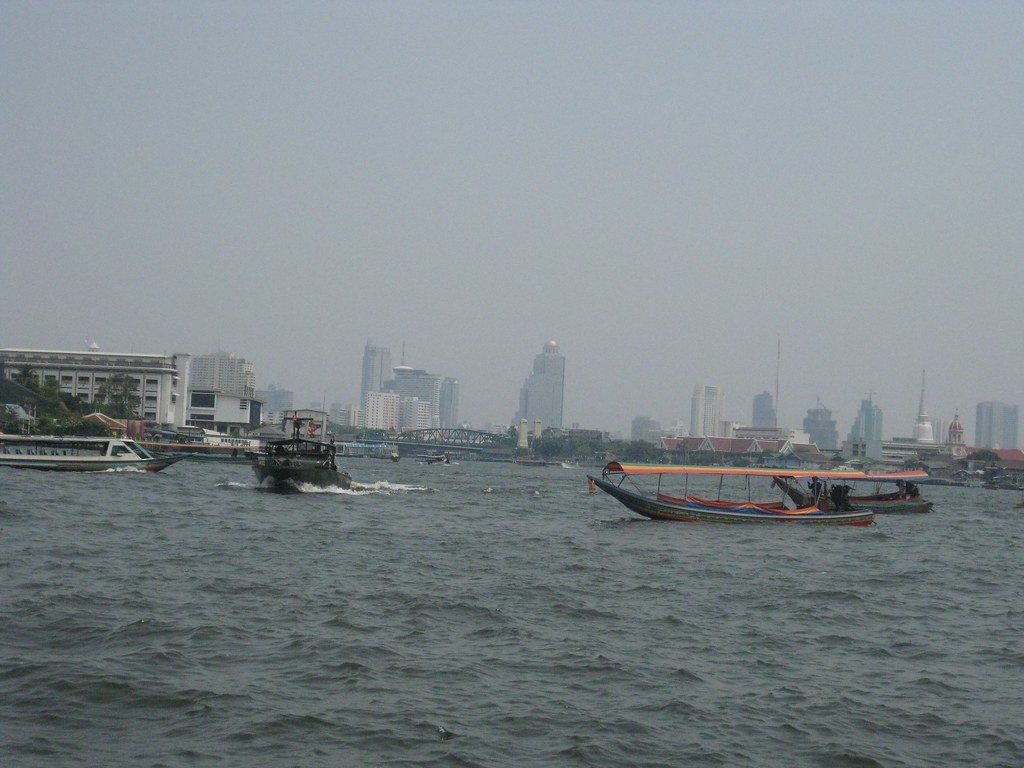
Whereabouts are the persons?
[807,477,822,497]
[894,479,919,497]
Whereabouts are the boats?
[256,408,353,491]
[427,460,432,464]
[392,453,399,462]
[772,470,934,517]
[588,459,881,526]
[136,426,260,462]
[1,433,197,475]
[415,455,447,463]
